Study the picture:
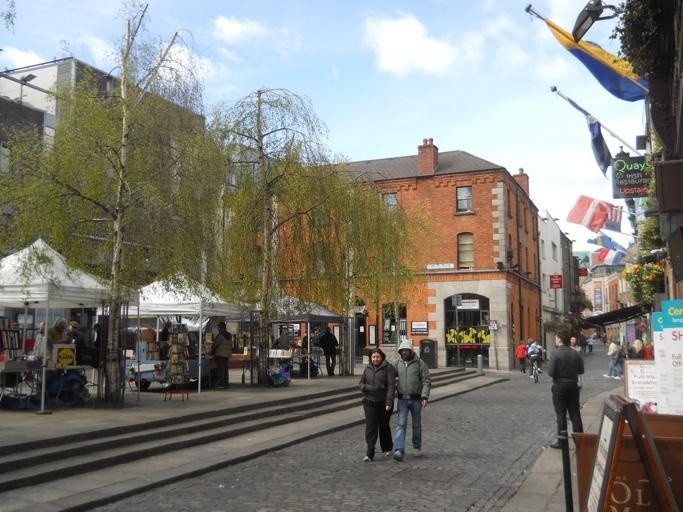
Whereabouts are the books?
[0,316,25,361]
[145,343,168,361]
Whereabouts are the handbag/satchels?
[533,347,541,355]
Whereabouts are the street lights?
[371,267,383,354]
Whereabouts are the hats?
[70,321,80,329]
[216,322,226,326]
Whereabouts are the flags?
[543,19,652,102]
[567,195,627,278]
[566,98,612,177]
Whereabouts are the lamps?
[572,0,626,43]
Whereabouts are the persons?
[515,341,528,374]
[586,336,596,351]
[47,318,67,355]
[265,327,339,376]
[91,321,102,345]
[32,320,46,360]
[210,321,233,391]
[159,320,172,338]
[388,338,431,461]
[525,338,545,380]
[548,331,585,450]
[601,336,653,382]
[357,348,396,463]
[66,320,87,346]
[567,336,584,388]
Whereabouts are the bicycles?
[526,351,544,382]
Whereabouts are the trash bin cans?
[420,339,435,369]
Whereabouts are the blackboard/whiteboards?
[580,398,621,512]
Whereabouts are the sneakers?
[363,448,421,461]
[603,373,623,380]
[215,384,224,389]
[551,441,560,449]
[530,368,542,378]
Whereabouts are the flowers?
[620,262,664,307]
